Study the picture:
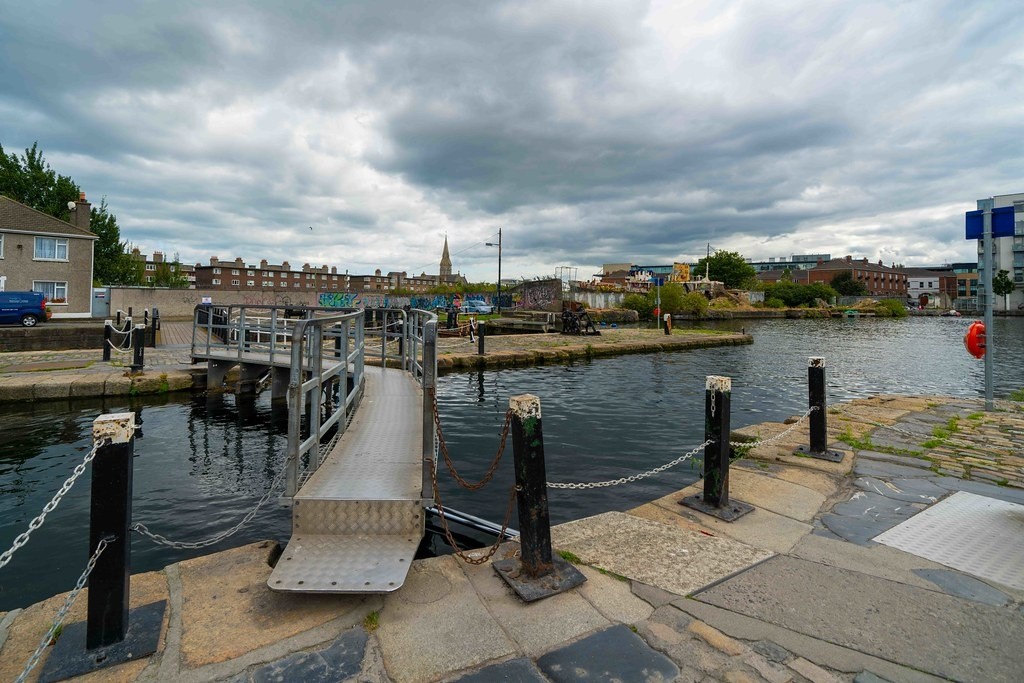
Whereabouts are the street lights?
[486,243,501,313]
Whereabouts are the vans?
[0,290,47,327]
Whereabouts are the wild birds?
[309,226,312,230]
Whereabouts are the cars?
[461,301,491,314]
